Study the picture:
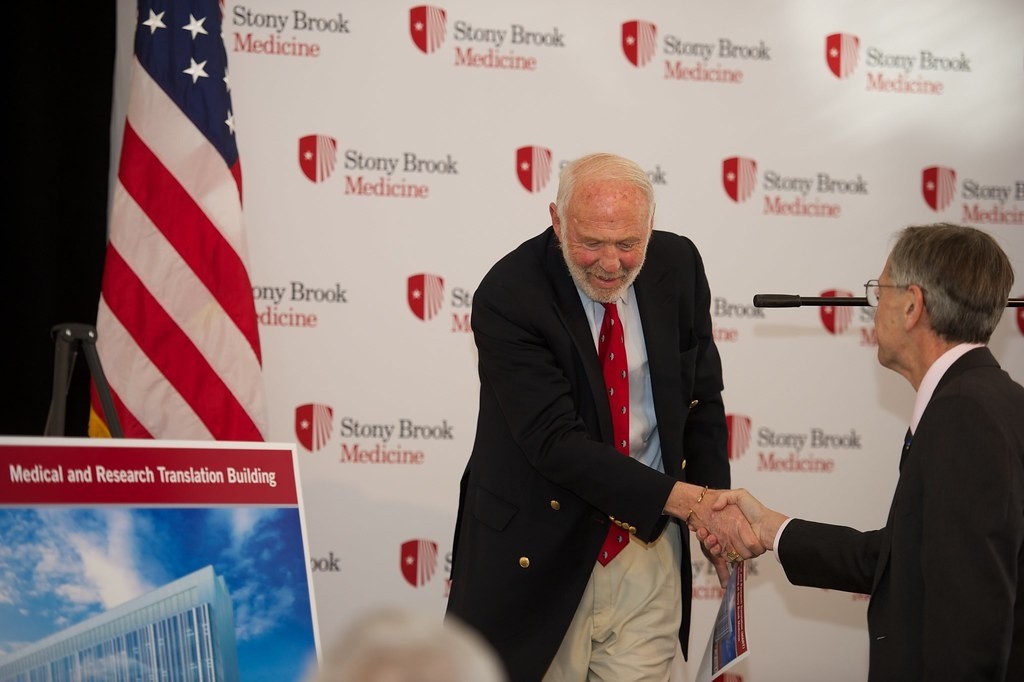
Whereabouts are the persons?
[687,223,1024,682]
[303,607,506,682]
[445,153,766,681]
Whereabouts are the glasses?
[863,279,928,308]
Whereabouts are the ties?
[596,302,631,566]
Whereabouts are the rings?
[727,549,739,560]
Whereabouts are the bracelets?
[685,485,708,524]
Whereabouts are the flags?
[89,0,270,441]
